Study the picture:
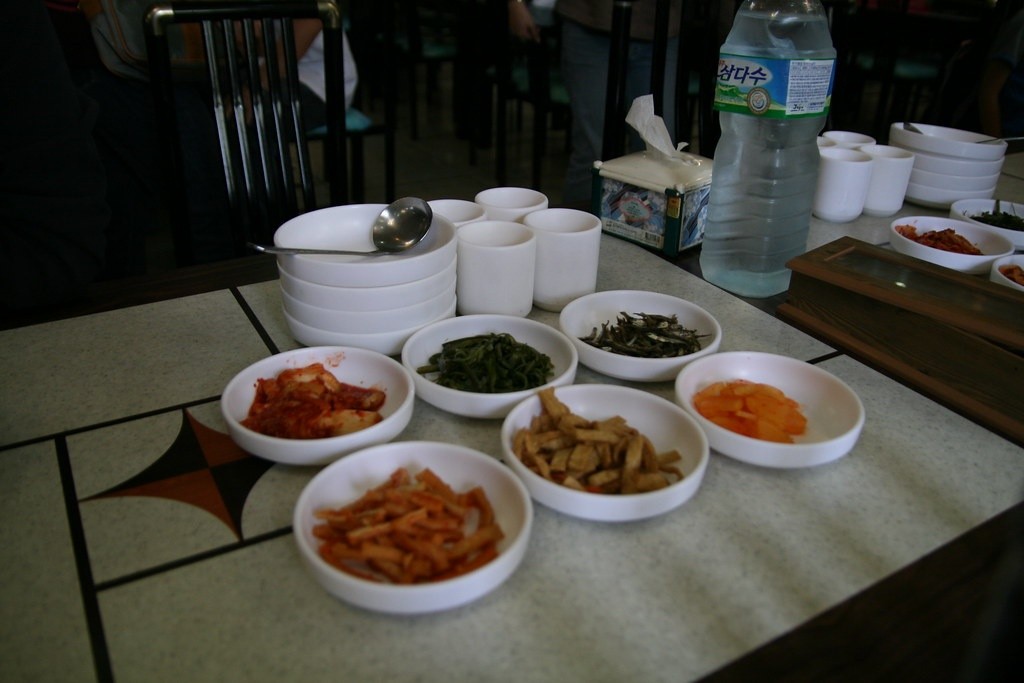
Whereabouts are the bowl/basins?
[221,345,416,466]
[559,289,722,382]
[273,204,458,356]
[675,350,864,466]
[951,199,1024,250]
[889,216,1015,274]
[502,383,708,520]
[990,254,1024,292]
[402,314,578,417]
[889,122,1008,209]
[293,441,534,613]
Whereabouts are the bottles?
[699,0,837,298]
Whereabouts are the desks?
[0,231,1024,683]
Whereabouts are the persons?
[961,0,1023,139]
[508,0,734,217]
[209,1,360,257]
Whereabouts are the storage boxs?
[775,235,1024,448]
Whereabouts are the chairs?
[141,0,1024,268]
[805,152,1024,255]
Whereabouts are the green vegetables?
[963,200,1024,233]
[416,332,554,393]
[578,310,714,357]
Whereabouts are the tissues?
[590,93,716,255]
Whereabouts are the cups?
[813,131,876,222]
[475,187,602,312]
[428,200,535,318]
[858,145,915,218]
